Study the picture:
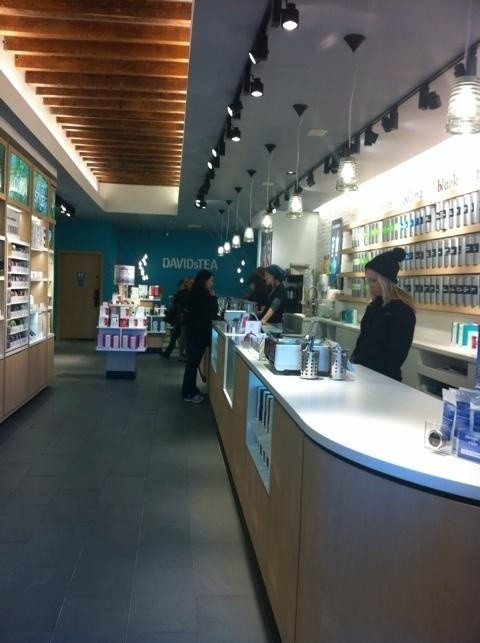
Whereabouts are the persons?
[347,246,416,380]
[246,268,261,302]
[179,268,220,403]
[162,277,195,363]
[261,267,290,322]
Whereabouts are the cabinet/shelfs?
[335,178,480,316]
[0,136,59,424]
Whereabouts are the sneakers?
[184,395,204,403]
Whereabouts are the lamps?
[217,33,366,257]
[446,1,480,135]
[362,58,466,149]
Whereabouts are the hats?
[364,247,406,283]
[263,265,285,280]
